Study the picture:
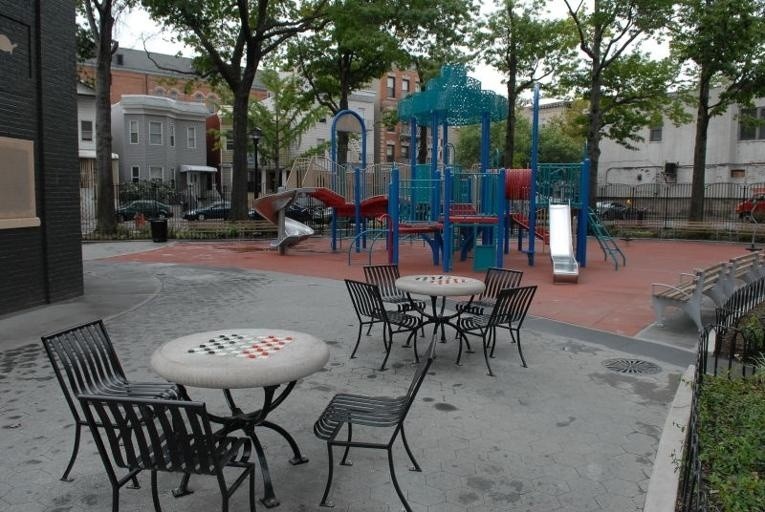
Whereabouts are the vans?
[736,192,765,223]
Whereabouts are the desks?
[395,275,486,353]
[150,328,330,509]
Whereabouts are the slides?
[252,189,315,250]
[547,198,579,282]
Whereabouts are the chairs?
[344,279,421,371]
[456,285,537,376]
[79,394,256,512]
[41,319,193,489]
[363,264,426,343]
[314,334,437,512]
[455,267,523,348]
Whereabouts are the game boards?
[189,330,246,358]
[415,274,464,284]
[242,335,293,358]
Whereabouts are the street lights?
[249,127,262,200]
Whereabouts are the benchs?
[651,262,728,337]
[693,251,761,298]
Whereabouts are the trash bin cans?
[148,217,168,243]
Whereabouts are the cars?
[183,200,232,222]
[596,201,647,220]
[247,203,309,223]
[311,206,333,224]
[114,198,172,222]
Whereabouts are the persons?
[134,210,146,229]
